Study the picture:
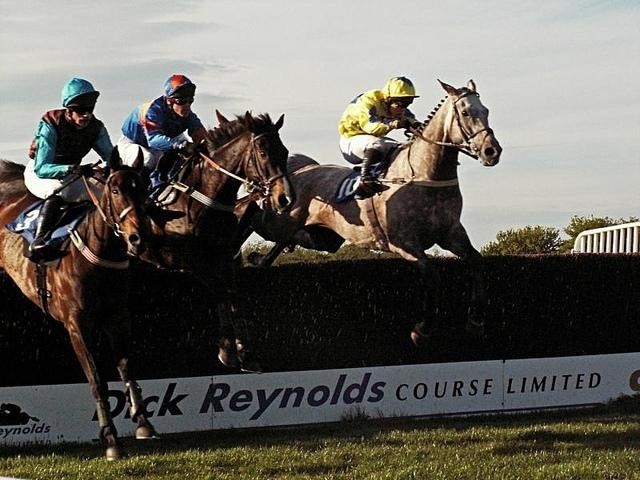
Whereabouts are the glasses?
[67,105,95,114]
[391,98,413,109]
[173,98,194,105]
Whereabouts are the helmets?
[62,77,100,107]
[386,76,420,98]
[164,75,196,96]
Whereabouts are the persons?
[336,76,424,195]
[114,73,207,194]
[21,77,124,262]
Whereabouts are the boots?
[28,196,60,262]
[361,157,376,185]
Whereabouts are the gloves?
[173,140,194,159]
[397,119,411,129]
[412,122,423,129]
[71,164,95,177]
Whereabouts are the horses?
[142,109,297,373]
[0,144,163,462]
[247,77,502,348]
